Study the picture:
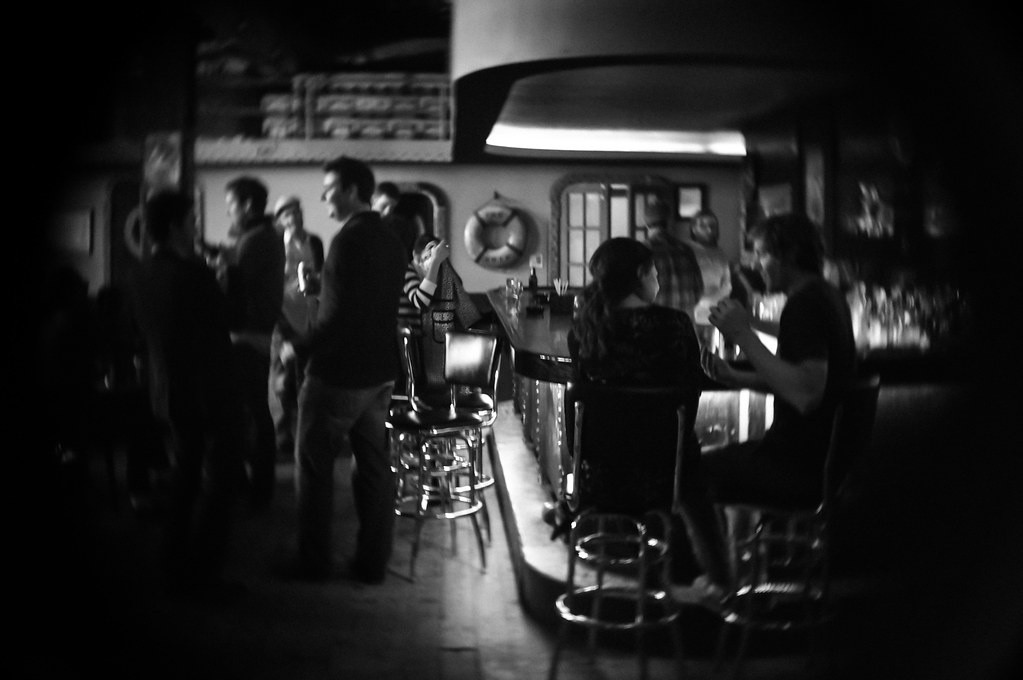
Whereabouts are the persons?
[0,158,487,680]
[543,207,860,610]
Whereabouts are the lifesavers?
[462,205,528,268]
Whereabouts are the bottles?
[529,268,537,288]
[848,280,934,349]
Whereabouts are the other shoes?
[335,562,388,586]
[693,575,736,610]
[277,563,332,586]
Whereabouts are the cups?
[505,278,523,312]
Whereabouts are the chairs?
[376,317,883,680]
